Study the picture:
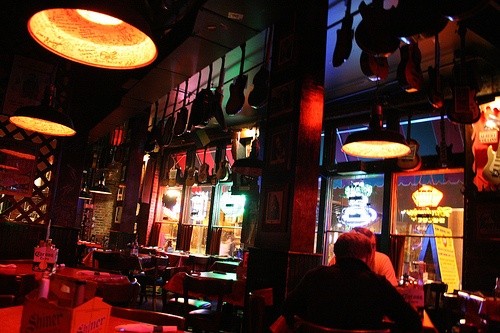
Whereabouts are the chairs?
[181,273,235,333]
[93,250,129,277]
[0,274,37,307]
[289,314,391,333]
[138,254,169,309]
[417,281,447,333]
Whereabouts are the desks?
[0,249,243,333]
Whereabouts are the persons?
[283,232,422,333]
[329,227,398,286]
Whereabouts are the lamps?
[90,154,112,194]
[28,0,171,70]
[341,95,414,159]
[78,175,92,199]
[10,86,77,137]
